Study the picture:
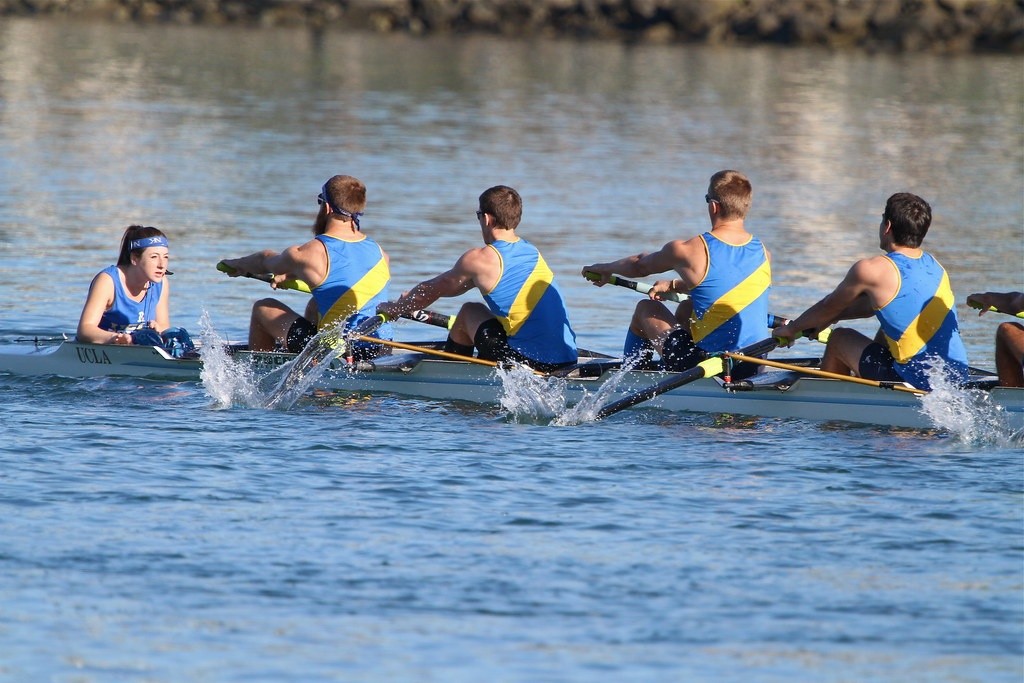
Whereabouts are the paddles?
[582,327,816,422]
[582,270,998,376]
[284,311,392,392]
[969,299,1024,320]
[214,262,618,361]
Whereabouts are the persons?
[375,184,579,378]
[582,170,773,383]
[221,174,394,361]
[74,225,174,345]
[967,291,1024,388]
[771,191,970,393]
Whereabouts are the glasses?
[317,194,333,213]
[476,210,490,220]
[705,194,720,204]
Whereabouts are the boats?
[0,327,1023,443]
